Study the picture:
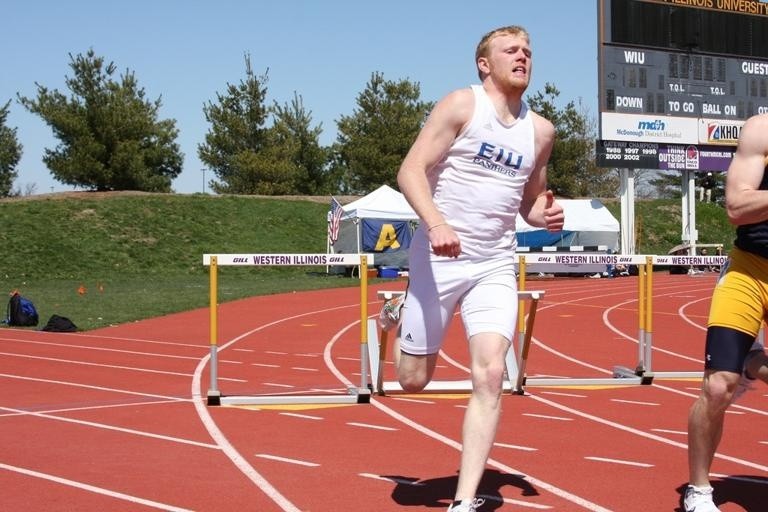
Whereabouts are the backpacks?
[5,291,39,328]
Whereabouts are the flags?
[328,195,345,246]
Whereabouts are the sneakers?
[444,497,485,512]
[728,339,764,405]
[376,293,407,332]
[683,483,720,511]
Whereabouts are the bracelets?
[426,222,450,230]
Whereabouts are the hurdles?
[201,253,731,406]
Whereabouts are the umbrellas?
[668,244,693,252]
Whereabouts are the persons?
[670,249,689,274]
[682,113,768,511]
[698,247,712,271]
[378,24,565,511]
